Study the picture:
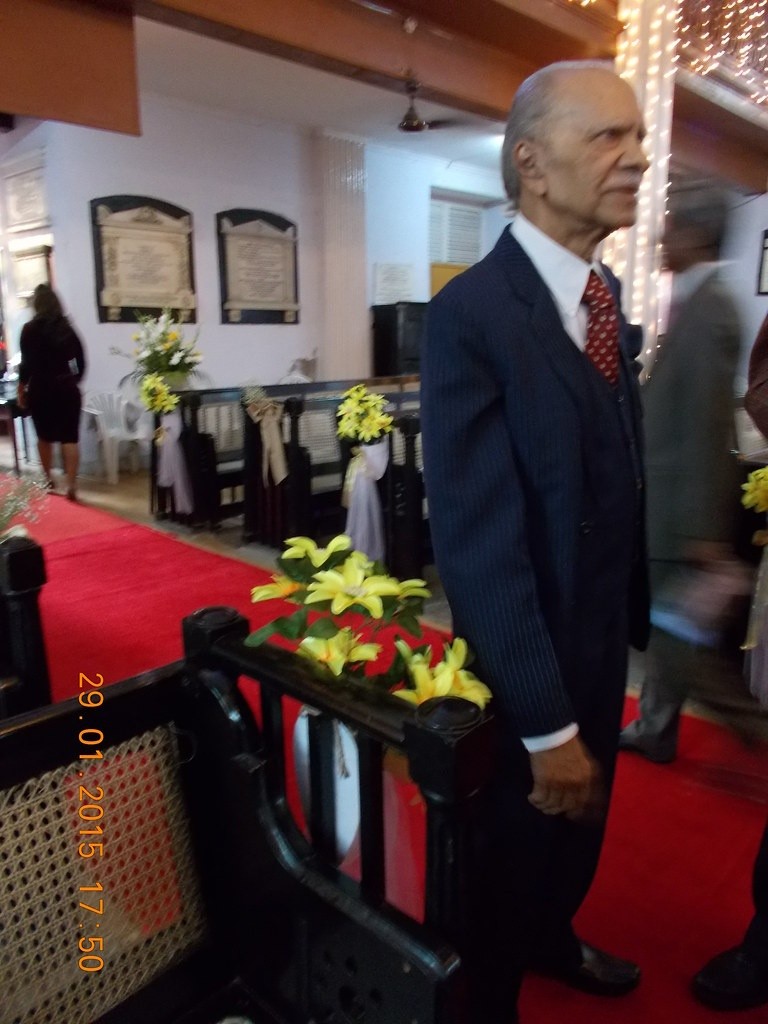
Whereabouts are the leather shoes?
[694,942,768,1010]
[526,941,641,995]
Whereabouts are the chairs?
[81,386,156,484]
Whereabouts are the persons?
[419,62,660,1024]
[618,183,768,1007]
[17,285,85,500]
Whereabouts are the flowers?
[140,372,181,416]
[333,382,392,445]
[246,535,493,709]
[740,466,768,548]
[108,304,210,392]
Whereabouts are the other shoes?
[618,729,675,763]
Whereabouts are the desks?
[0,394,35,476]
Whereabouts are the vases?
[291,703,357,862]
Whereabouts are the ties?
[581,270,619,395]
